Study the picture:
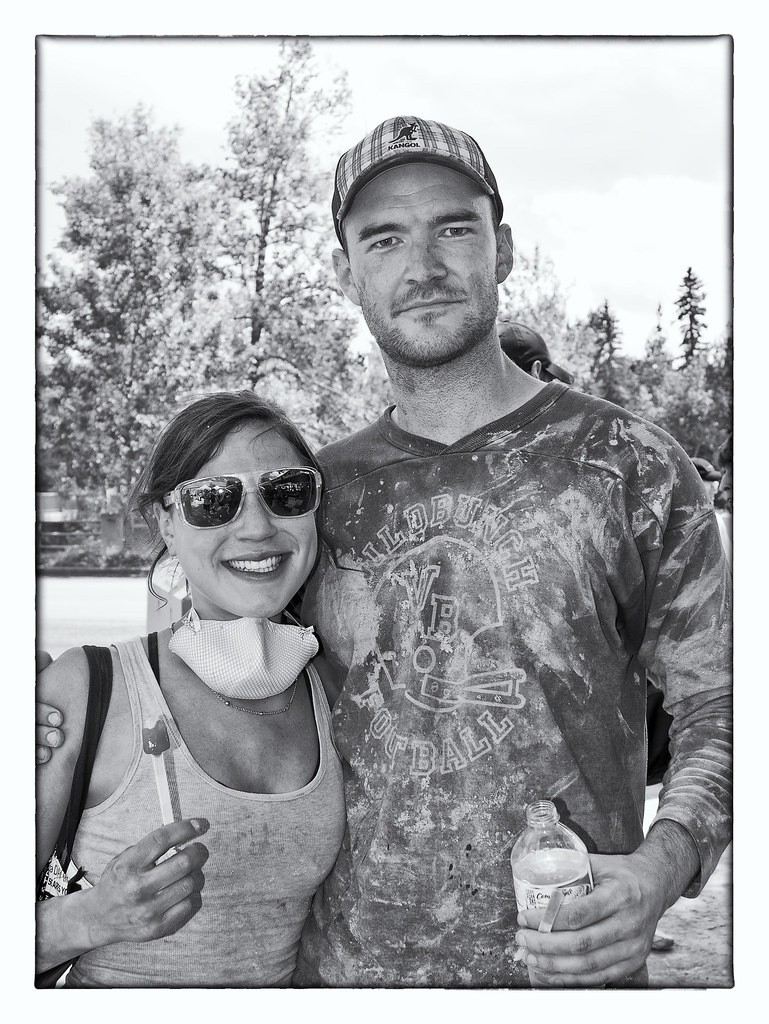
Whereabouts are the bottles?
[509,798,595,990]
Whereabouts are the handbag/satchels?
[36,644,114,989]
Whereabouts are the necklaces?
[170,622,299,716]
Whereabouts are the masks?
[168,608,319,699]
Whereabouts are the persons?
[498,319,575,385]
[35,114,735,990]
[35,389,347,989]
[713,430,733,540]
[689,457,722,507]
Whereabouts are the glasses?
[162,467,323,529]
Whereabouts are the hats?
[331,116,504,248]
[691,456,722,482]
[496,319,575,385]
